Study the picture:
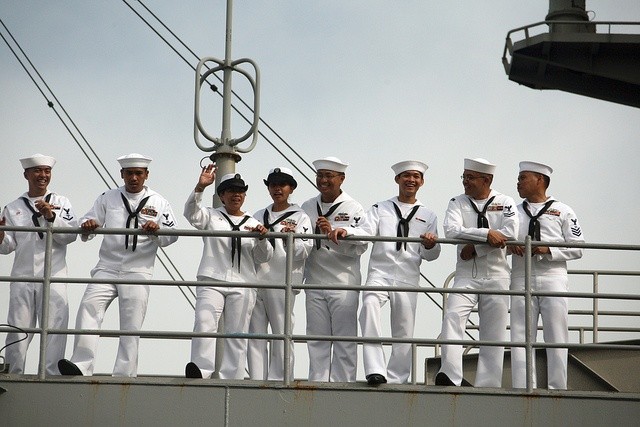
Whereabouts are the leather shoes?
[435,372,454,385]
[186,362,202,380]
[366,373,386,383]
[58,359,82,376]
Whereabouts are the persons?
[247,166,314,381]
[58,153,179,377]
[327,160,441,385]
[435,156,520,388]
[300,157,368,383]
[510,160,585,389]
[0,154,78,376]
[183,163,273,380]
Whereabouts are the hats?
[216,173,249,194]
[263,167,298,189]
[312,156,348,173]
[519,161,553,179]
[464,157,496,174]
[117,153,152,169]
[391,159,428,175]
[19,154,56,170]
